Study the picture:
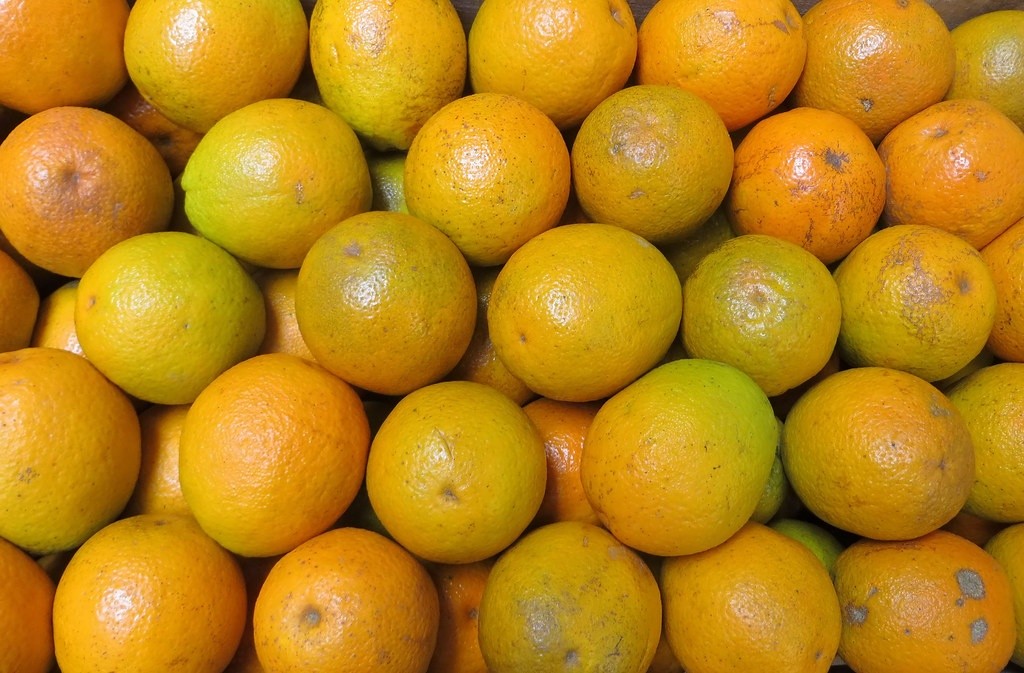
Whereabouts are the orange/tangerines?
[0,0,1024,673]
